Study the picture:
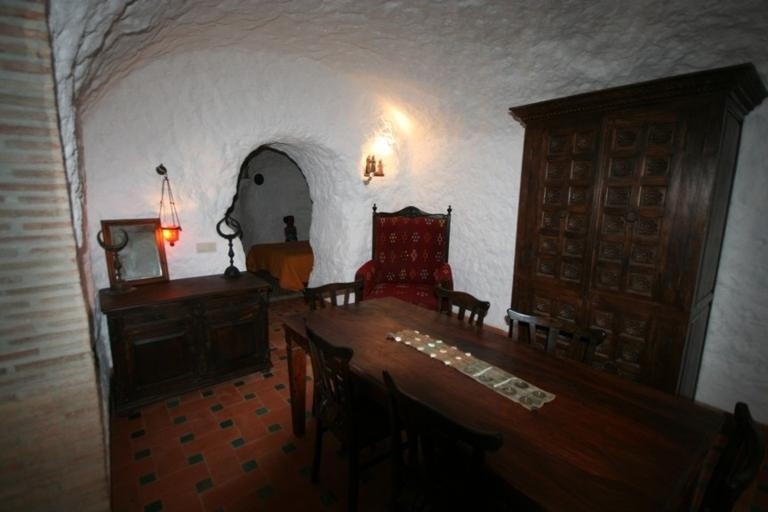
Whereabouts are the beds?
[246,241,313,294]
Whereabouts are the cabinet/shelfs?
[97,271,278,413]
[509,61,768,401]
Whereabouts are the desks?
[280,294,768,511]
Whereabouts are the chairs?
[353,203,454,313]
[432,285,492,324]
[381,370,506,512]
[506,308,607,367]
[302,274,366,418]
[303,325,385,511]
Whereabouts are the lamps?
[157,165,183,248]
[363,155,385,185]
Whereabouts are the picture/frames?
[101,218,171,289]
